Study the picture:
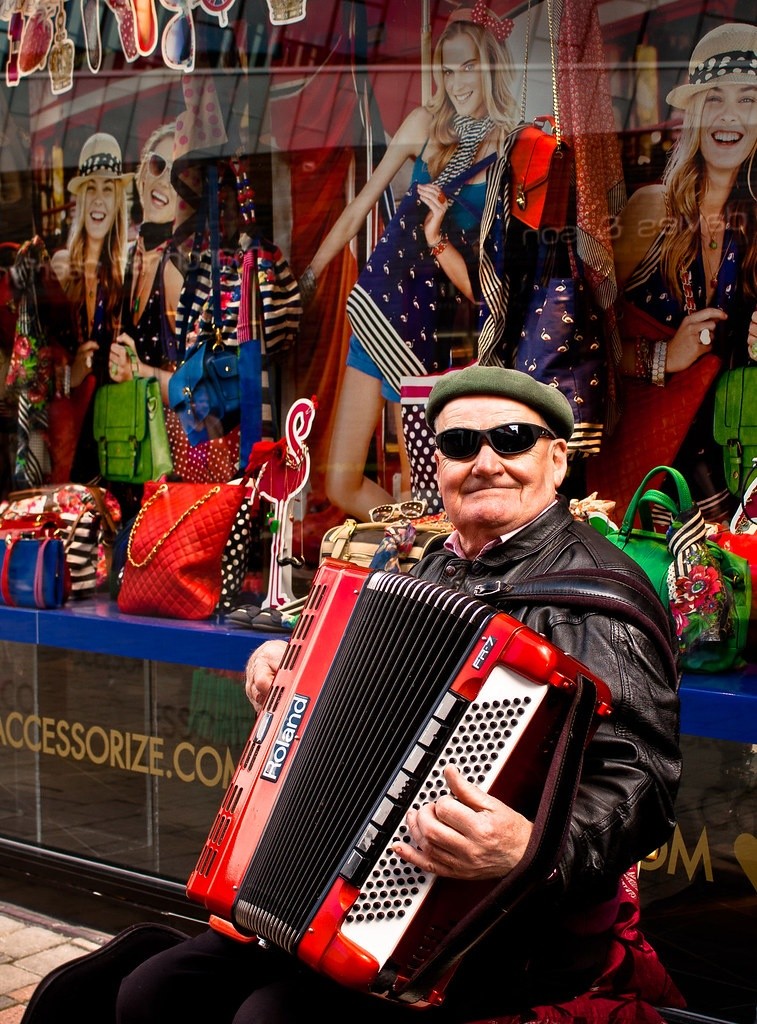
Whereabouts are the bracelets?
[64,367,70,394]
[428,230,448,255]
[300,266,318,294]
[634,337,668,387]
[154,366,161,386]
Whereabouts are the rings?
[700,329,711,345]
[111,363,116,375]
[86,356,92,368]
[438,192,445,204]
[751,339,757,357]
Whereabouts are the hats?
[666,24,757,110]
[66,132,136,195]
[424,366,573,445]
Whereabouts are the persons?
[23,366,684,1024]
[53,133,130,399]
[105,126,187,404]
[611,23,757,532]
[297,8,520,522]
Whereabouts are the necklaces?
[133,259,156,311]
[699,209,733,288]
[86,276,97,325]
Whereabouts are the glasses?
[147,150,175,178]
[368,500,426,523]
[435,422,556,461]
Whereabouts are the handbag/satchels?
[168,339,242,447]
[175,154,302,356]
[583,186,721,532]
[319,518,455,575]
[603,464,750,675]
[345,152,510,395]
[713,357,757,497]
[0,346,97,488]
[0,483,125,609]
[92,342,173,483]
[708,471,757,615]
[117,479,254,622]
[508,112,571,234]
[517,226,608,456]
